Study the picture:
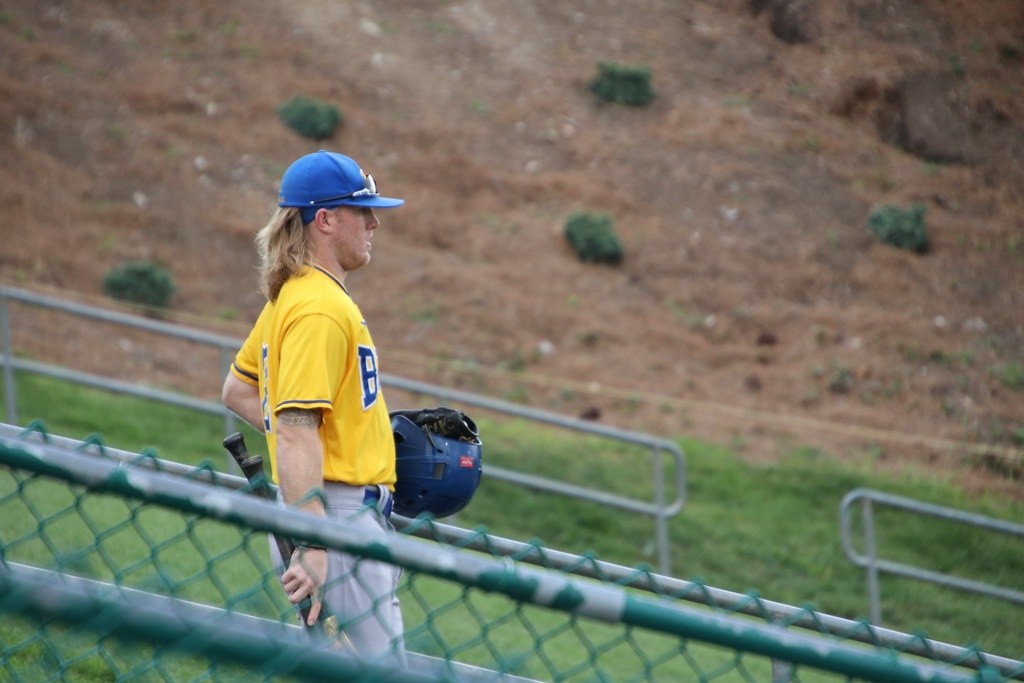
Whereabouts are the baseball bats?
[222,431,360,663]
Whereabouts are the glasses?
[310,173,379,204]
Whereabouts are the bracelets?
[292,539,327,551]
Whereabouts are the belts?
[364,487,395,518]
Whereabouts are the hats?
[277,149,406,209]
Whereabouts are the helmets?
[389,408,483,520]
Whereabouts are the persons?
[220,150,407,667]
